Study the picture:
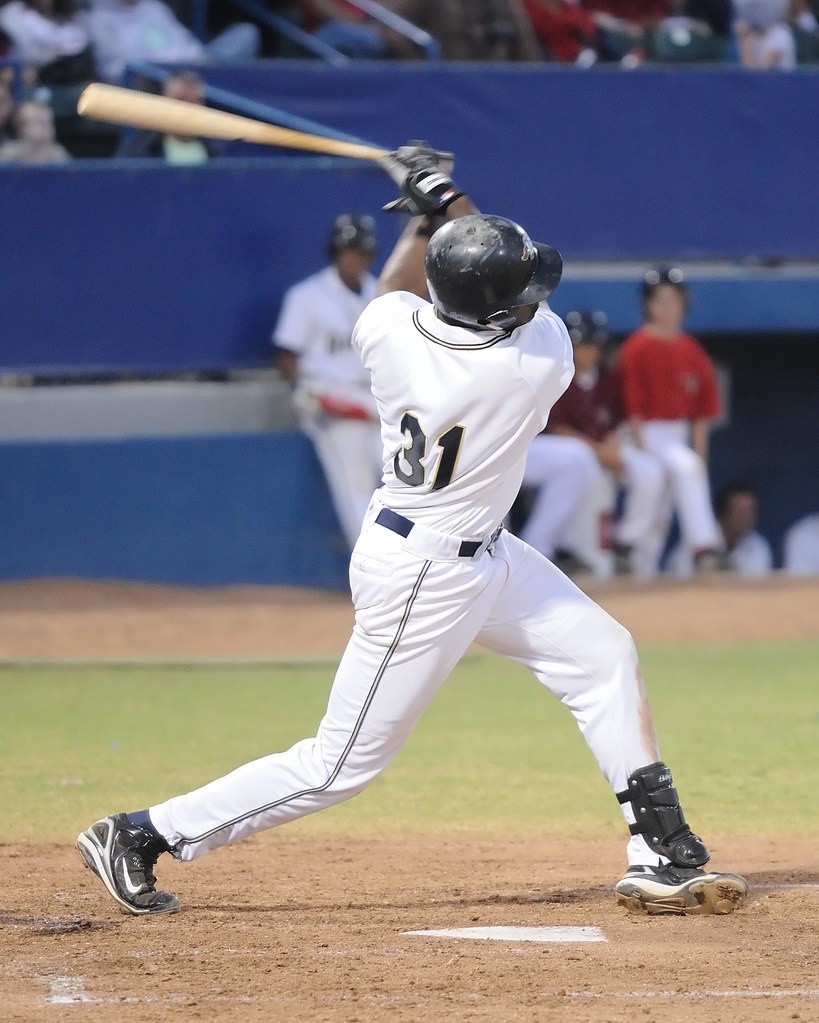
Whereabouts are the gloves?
[385,148,469,223]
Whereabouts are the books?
[300,0,819,70]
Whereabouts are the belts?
[372,508,483,560]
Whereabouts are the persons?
[0,0,260,166]
[270,213,386,550]
[500,268,819,577]
[76,146,750,917]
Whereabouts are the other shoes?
[695,551,737,573]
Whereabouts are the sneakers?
[75,811,181,917]
[616,865,747,917]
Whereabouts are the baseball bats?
[75,81,446,164]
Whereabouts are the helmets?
[642,266,686,295]
[327,213,379,255]
[562,310,612,349]
[423,211,566,326]
